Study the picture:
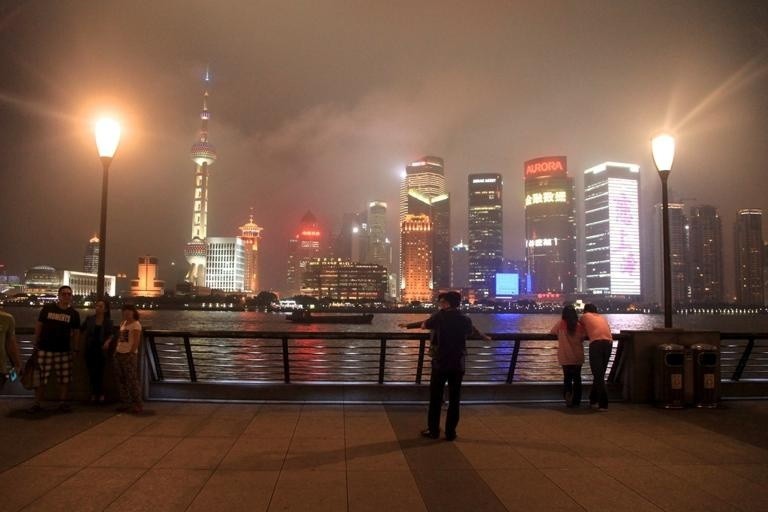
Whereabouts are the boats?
[284,311,375,324]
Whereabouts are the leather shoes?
[418,423,460,441]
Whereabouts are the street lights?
[649,130,677,329]
[92,115,122,298]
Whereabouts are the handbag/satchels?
[19,348,42,390]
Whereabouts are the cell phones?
[9,368,17,384]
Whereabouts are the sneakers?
[561,390,611,412]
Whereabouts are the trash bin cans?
[692,344,722,409]
[660,344,686,411]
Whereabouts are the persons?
[0,310,25,382]
[116,303,146,413]
[419,292,492,442]
[72,298,114,403]
[549,302,590,411]
[25,285,83,414]
[578,301,614,410]
[396,292,450,406]
